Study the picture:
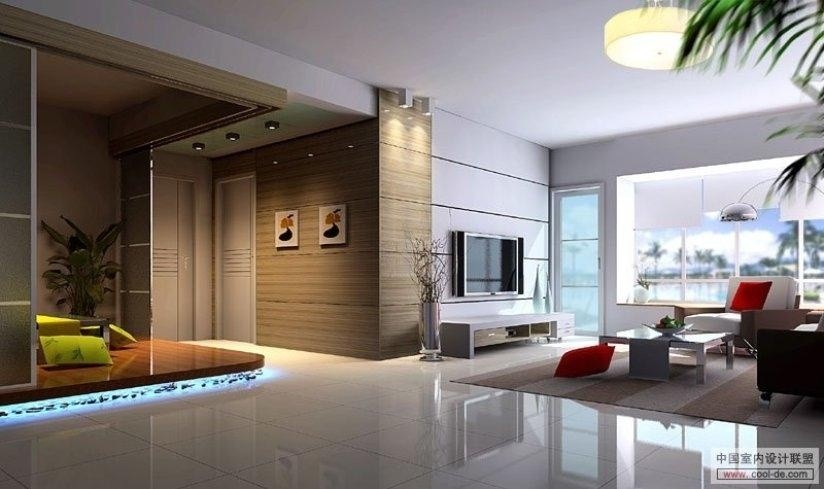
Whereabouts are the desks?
[612,295,725,329]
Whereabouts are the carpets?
[447,337,810,430]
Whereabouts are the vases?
[545,277,554,315]
[414,299,444,363]
[530,264,542,315]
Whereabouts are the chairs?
[670,274,801,358]
[753,309,824,406]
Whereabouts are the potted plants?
[631,252,659,305]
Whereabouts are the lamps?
[600,0,720,73]
[190,120,280,152]
[420,96,433,116]
[396,87,413,109]
[716,173,823,223]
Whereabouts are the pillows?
[76,323,138,351]
[549,341,617,380]
[36,331,115,370]
[724,279,773,312]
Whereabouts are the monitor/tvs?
[463,231,518,296]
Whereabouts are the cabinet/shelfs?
[434,310,575,361]
